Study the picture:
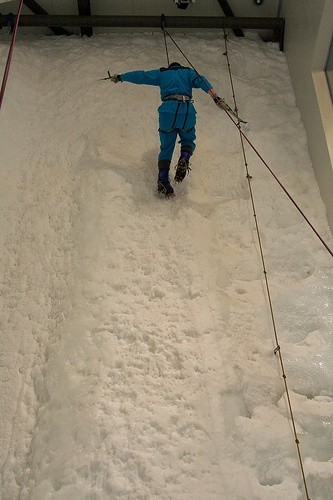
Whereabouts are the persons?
[111,62,231,193]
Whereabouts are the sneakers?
[175,155,188,181]
[158,168,174,195]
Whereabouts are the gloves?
[214,97,229,110]
[111,74,121,84]
